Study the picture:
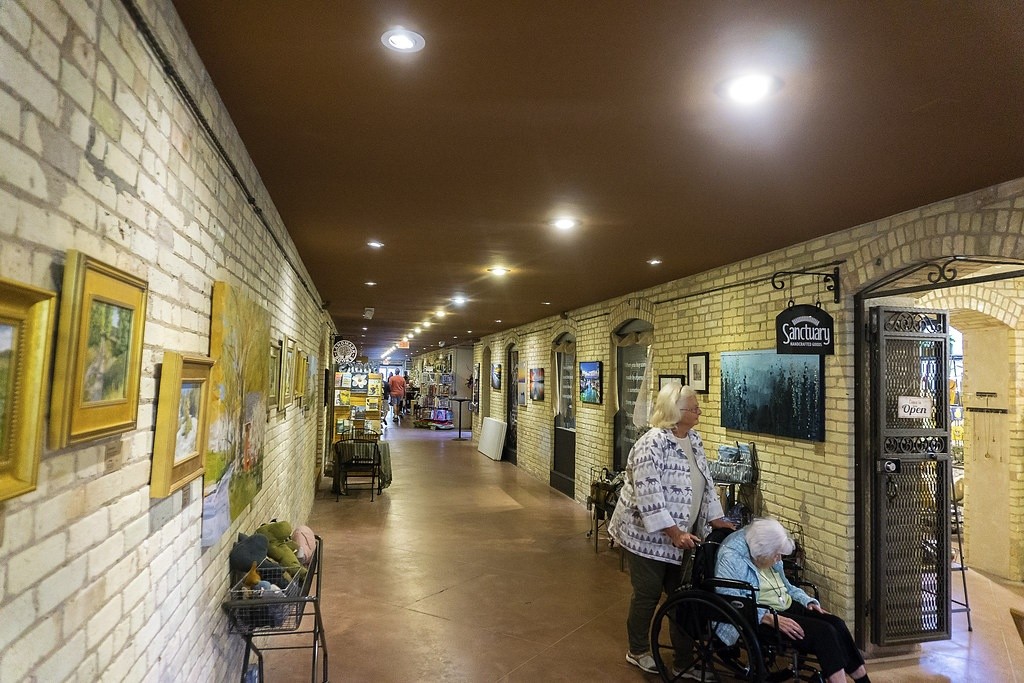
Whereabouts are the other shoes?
[399,415,403,418]
[393,418,399,422]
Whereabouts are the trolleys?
[584,465,624,550]
[221,535,328,683]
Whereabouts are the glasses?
[679,404,699,413]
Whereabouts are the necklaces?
[758,567,781,597]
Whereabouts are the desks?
[449,397,471,441]
[332,440,393,495]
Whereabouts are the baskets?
[227,566,303,626]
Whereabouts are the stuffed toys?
[230,517,316,626]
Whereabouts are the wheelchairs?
[649,538,826,683]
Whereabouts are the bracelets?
[810,600,818,605]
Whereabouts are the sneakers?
[672,664,721,682]
[626,650,667,674]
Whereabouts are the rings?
[793,632,796,634]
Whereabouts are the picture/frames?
[659,373,684,396]
[0,281,58,504]
[48,244,150,456]
[267,336,310,413]
[150,350,216,499]
[688,352,709,393]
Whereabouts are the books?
[334,372,381,435]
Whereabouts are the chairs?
[341,429,379,486]
[333,439,381,503]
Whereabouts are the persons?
[388,372,394,405]
[381,373,390,425]
[403,371,410,383]
[710,519,870,683]
[389,368,406,422]
[369,384,376,395]
[608,382,736,683]
